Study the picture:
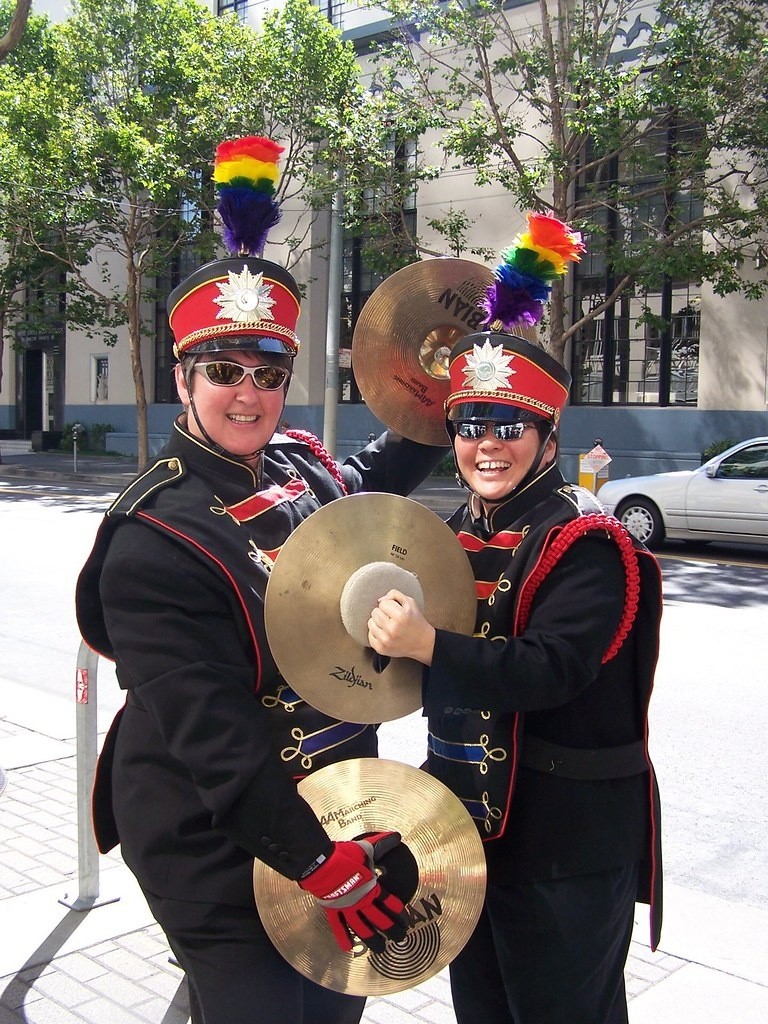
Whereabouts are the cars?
[596,437,768,552]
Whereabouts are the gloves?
[296,831,413,953]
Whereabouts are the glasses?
[193,360,291,391]
[451,421,549,440]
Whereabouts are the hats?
[166,136,302,361]
[443,211,587,429]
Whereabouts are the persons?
[368,332,665,1024]
[77,254,454,1024]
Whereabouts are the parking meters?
[593,439,602,496]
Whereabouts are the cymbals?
[351,256,538,448]
[252,757,488,998]
[263,491,478,726]
